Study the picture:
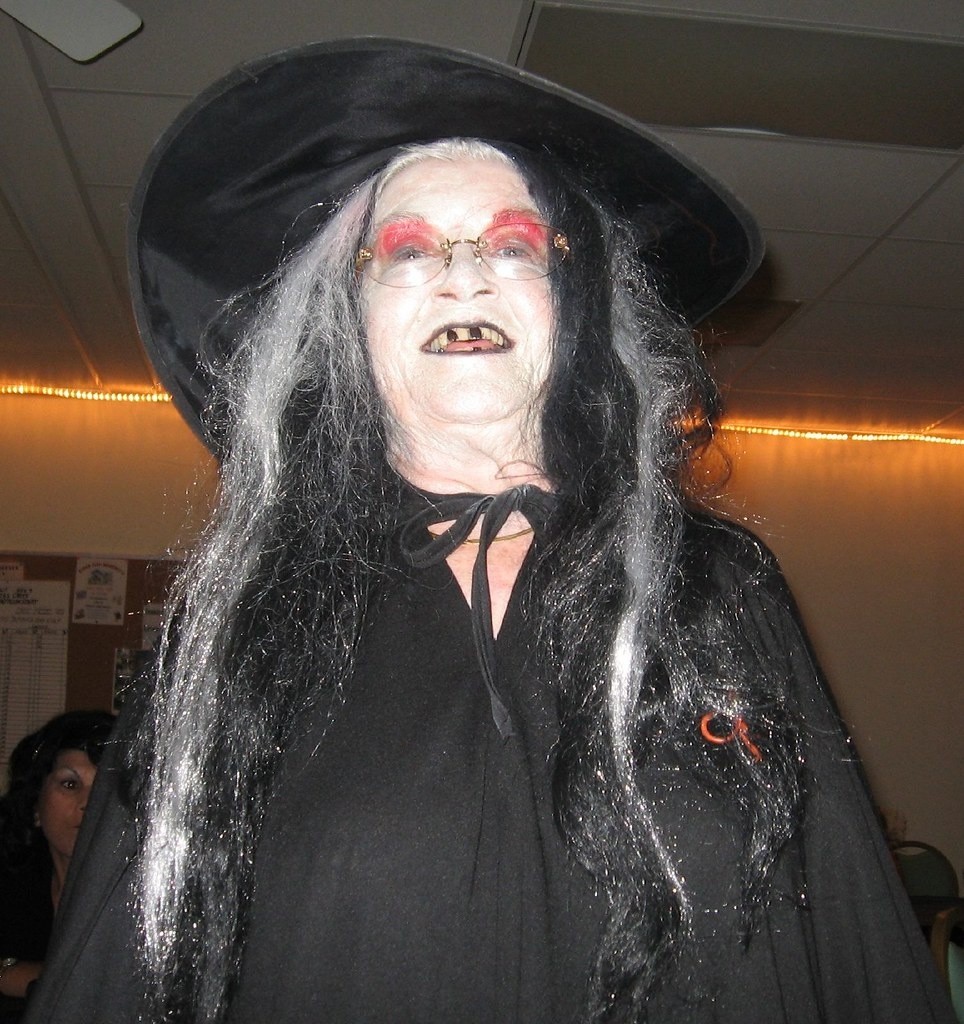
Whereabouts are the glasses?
[346,222,571,288]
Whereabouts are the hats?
[127,36,770,453]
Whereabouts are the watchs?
[0,957,17,979]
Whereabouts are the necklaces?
[430,528,532,545]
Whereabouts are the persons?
[0,709,120,1024]
[14,39,959,1024]
